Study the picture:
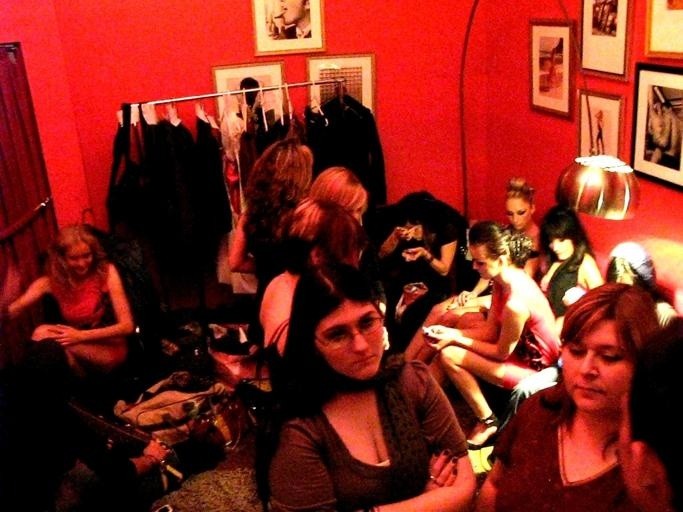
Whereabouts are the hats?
[611,242,656,287]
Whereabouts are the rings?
[430,475,437,481]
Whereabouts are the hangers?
[115,82,332,131]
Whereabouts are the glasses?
[316,302,384,349]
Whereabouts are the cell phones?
[423,326,435,335]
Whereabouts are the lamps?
[451,1,647,225]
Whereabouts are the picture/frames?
[249,0,328,59]
[211,60,287,126]
[644,0,683,60]
[577,0,634,85]
[524,16,579,124]
[307,50,379,117]
[575,88,627,165]
[627,62,682,192]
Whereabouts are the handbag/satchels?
[211,350,270,384]
[159,316,205,375]
[120,390,206,446]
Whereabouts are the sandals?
[469,412,501,450]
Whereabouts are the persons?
[1,257,169,511]
[229,140,367,389]
[254,261,475,512]
[9,227,132,366]
[475,282,659,512]
[620,317,682,511]
[376,178,678,448]
[596,110,605,155]
[647,85,683,169]
[266,0,311,41]
